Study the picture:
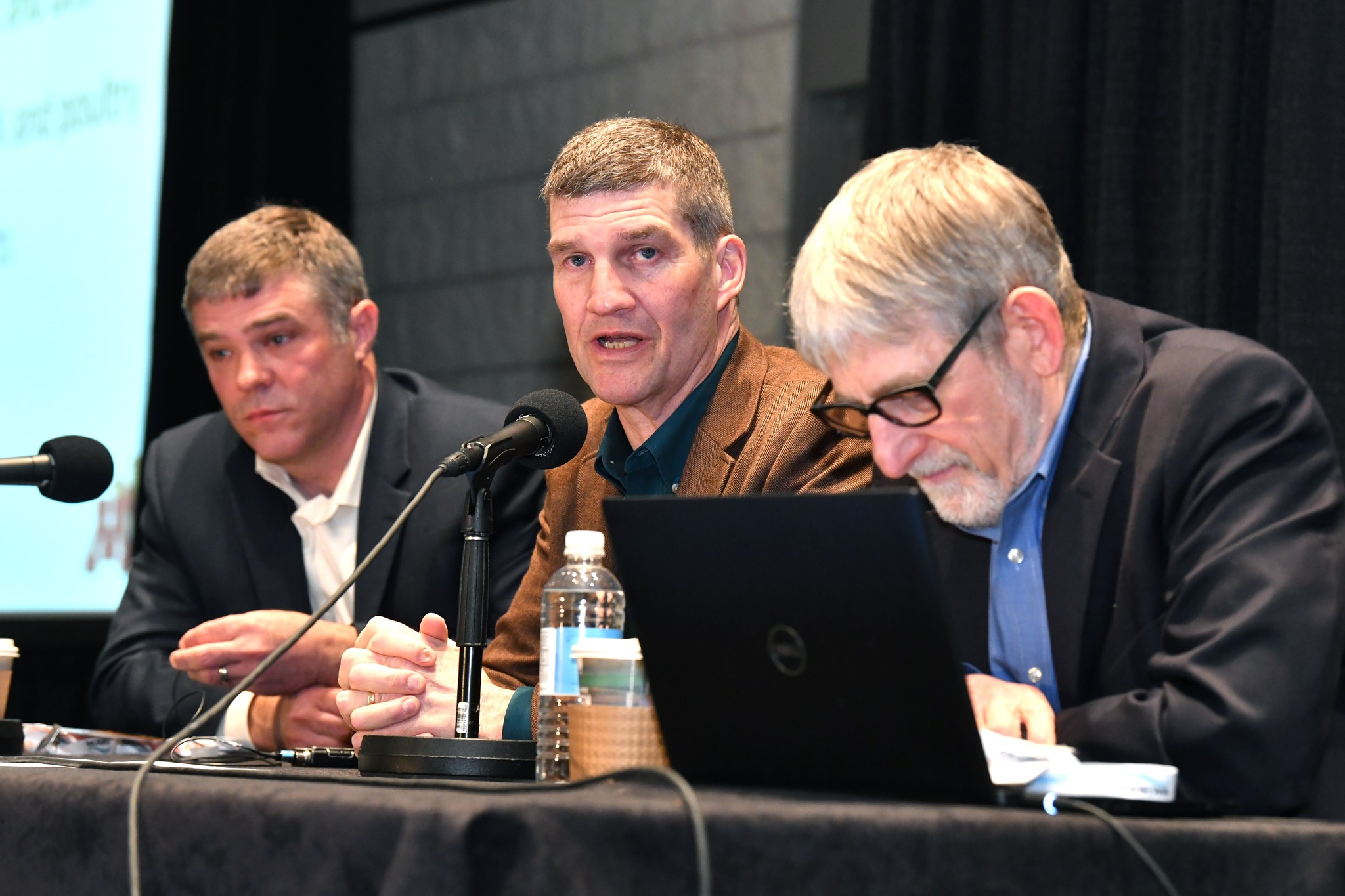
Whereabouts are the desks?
[0,742,1344,894]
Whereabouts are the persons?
[337,111,880,763]
[79,202,545,764]
[774,144,1342,818]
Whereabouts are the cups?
[566,632,670,786]
[1,634,20,725]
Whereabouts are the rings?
[218,664,232,685]
[363,691,375,704]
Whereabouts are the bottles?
[535,530,626,791]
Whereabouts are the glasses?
[809,293,1001,440]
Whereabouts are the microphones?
[439,388,587,477]
[0,435,114,503]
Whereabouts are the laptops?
[601,485,1242,817]
